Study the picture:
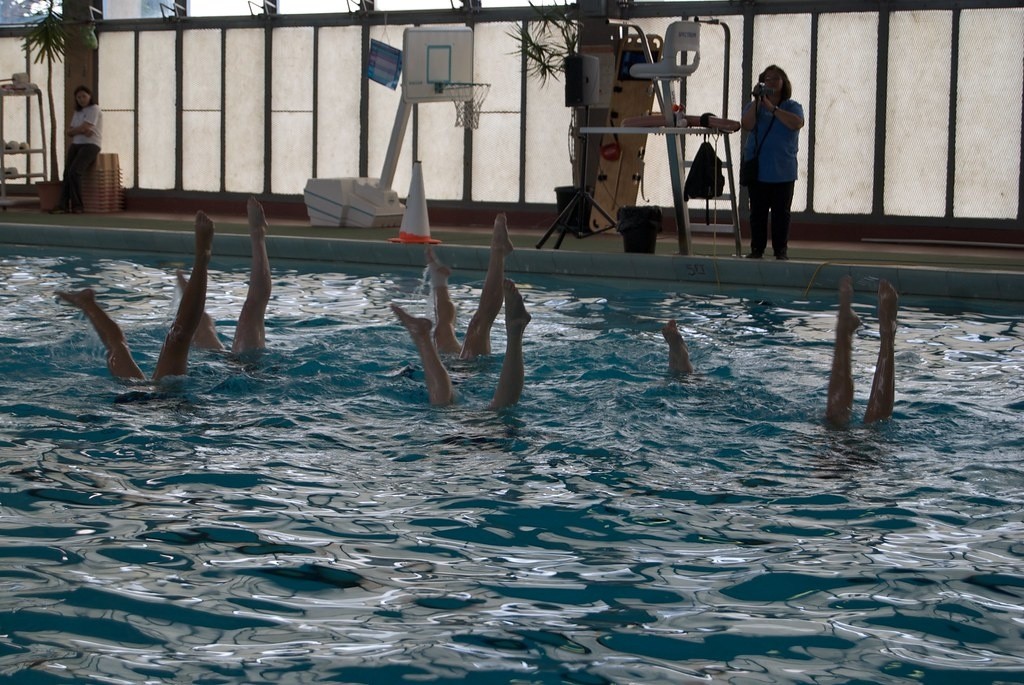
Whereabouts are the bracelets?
[770,106,778,113]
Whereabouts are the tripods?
[535,106,617,251]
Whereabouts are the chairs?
[630,21,702,127]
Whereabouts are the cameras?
[754,83,775,97]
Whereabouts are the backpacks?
[682,112,725,225]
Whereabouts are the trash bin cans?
[616,206,661,254]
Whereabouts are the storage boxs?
[80,151,124,213]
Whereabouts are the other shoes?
[48,205,72,214]
[775,255,789,259]
[745,252,765,258]
[70,208,82,213]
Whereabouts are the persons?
[391,277,531,407]
[826,275,898,421]
[425,212,514,358]
[176,196,271,354]
[740,65,805,260]
[52,210,214,379]
[45,86,103,213]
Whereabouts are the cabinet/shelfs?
[1,83,48,209]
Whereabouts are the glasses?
[763,76,778,82]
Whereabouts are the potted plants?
[502,0,589,230]
[20,9,86,212]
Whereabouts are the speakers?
[562,54,600,107]
